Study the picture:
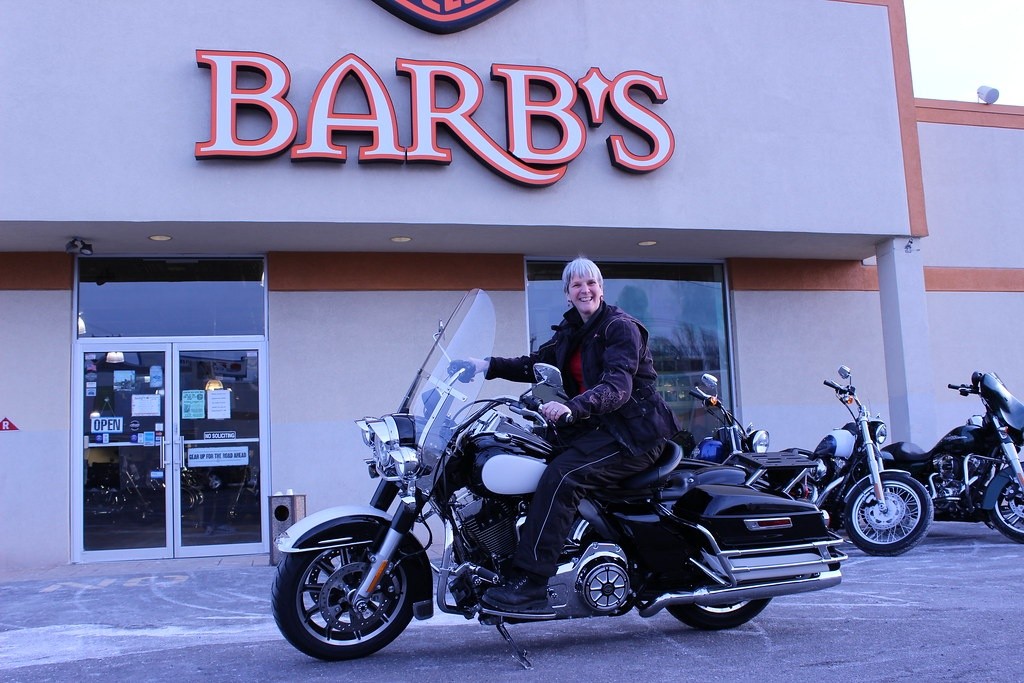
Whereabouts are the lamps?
[78,313,86,335]
[978,86,1000,104]
[106,352,125,363]
[205,308,223,391]
[66,238,93,256]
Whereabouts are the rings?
[553,409,557,413]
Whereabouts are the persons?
[198,419,238,537]
[463,252,685,613]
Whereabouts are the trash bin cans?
[268,495,306,566]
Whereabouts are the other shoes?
[482,569,549,612]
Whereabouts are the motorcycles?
[682,365,935,561]
[875,370,1024,542]
[266,287,848,672]
[82,451,260,530]
